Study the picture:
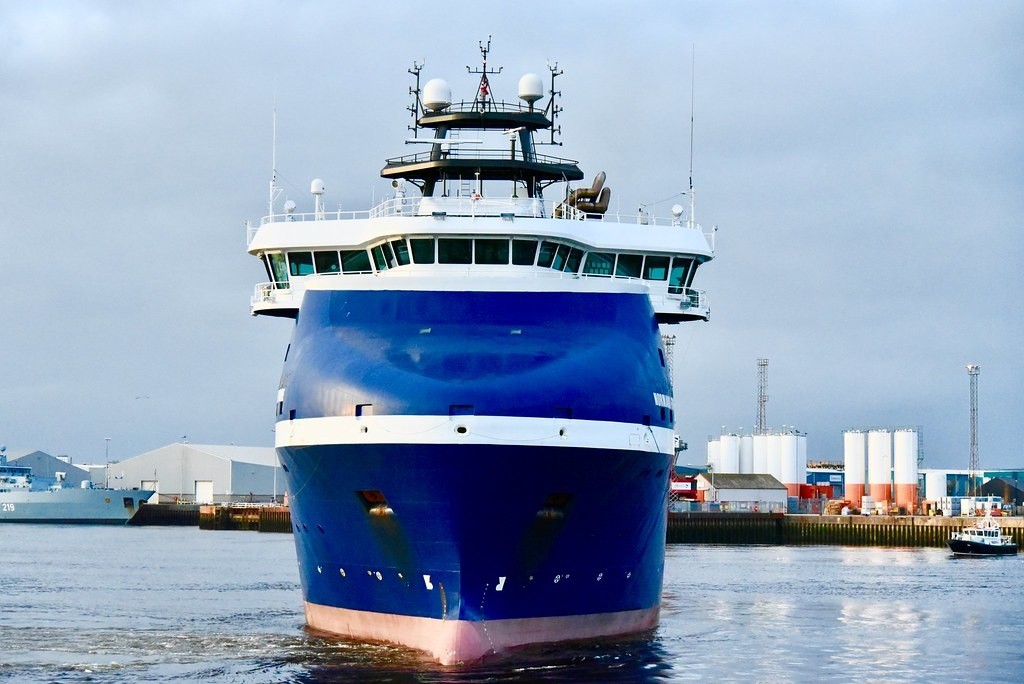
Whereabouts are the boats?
[0,444,156,526]
[245,33,721,674]
[944,493,1016,558]
